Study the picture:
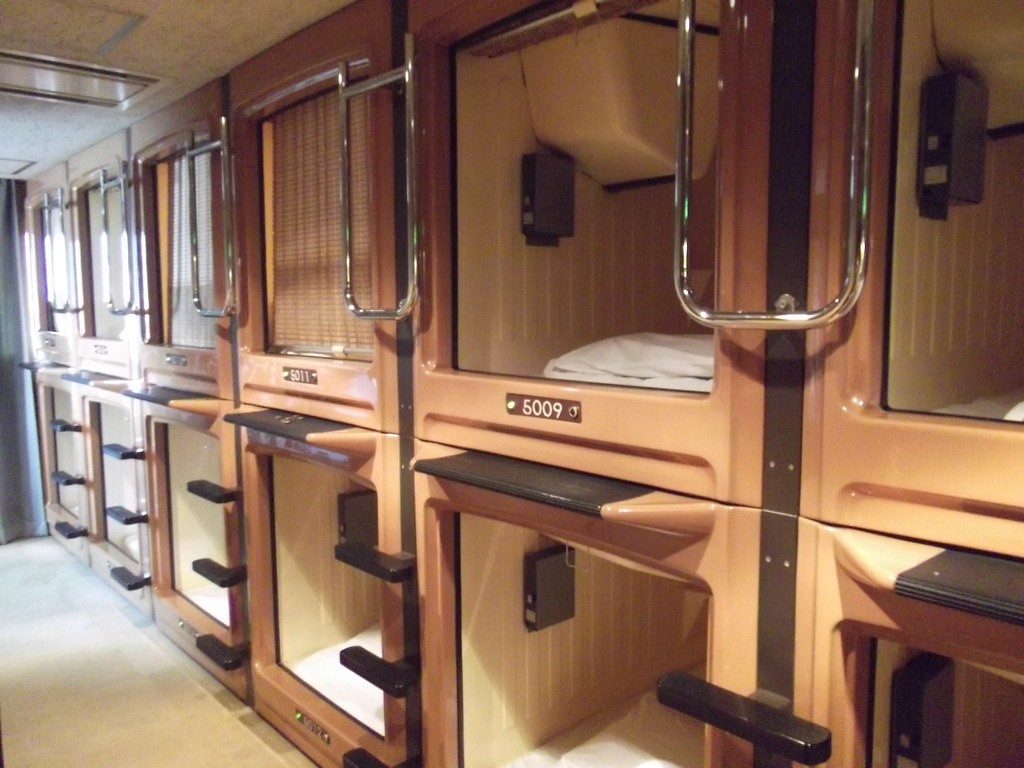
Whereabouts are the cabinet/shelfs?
[18,1,1024,768]
